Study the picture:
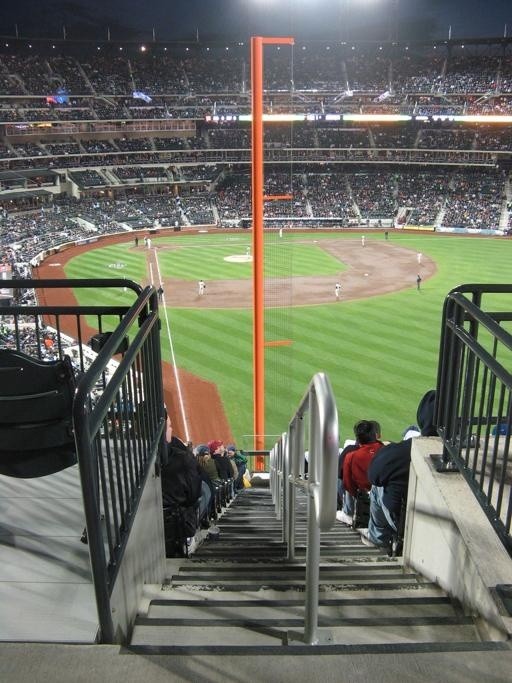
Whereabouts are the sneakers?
[183,521,220,555]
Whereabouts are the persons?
[158,285,163,301]
[335,282,341,298]
[248,246,251,259]
[417,275,421,289]
[131,438,248,537]
[361,236,365,246]
[199,280,205,295]
[135,237,138,245]
[0,116,511,164]
[303,438,440,549]
[1,262,144,437]
[417,251,422,264]
[2,164,511,267]
[280,228,282,237]
[143,235,151,248]
[0,49,511,131]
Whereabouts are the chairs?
[166,474,239,556]
[0,342,107,544]
[337,478,407,554]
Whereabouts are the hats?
[208,439,223,451]
[226,446,235,451]
[196,445,210,454]
